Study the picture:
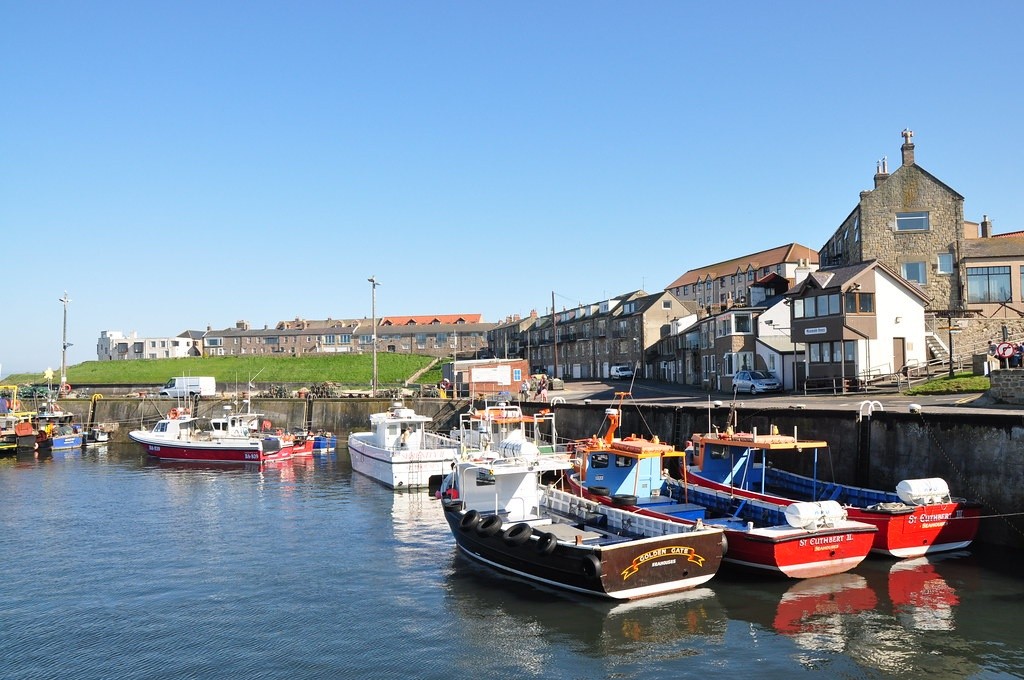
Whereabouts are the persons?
[988,341,1024,368]
[522,374,548,403]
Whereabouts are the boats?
[0,380,119,453]
[128,369,337,466]
[450,399,574,458]
[347,408,462,490]
[565,391,878,579]
[678,395,984,558]
[439,442,723,601]
[862,551,978,632]
[713,570,881,655]
[445,554,727,661]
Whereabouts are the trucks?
[611,366,634,381]
[159,377,216,399]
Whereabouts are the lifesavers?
[64,383,72,393]
[169,407,180,419]
[503,522,533,545]
[460,509,480,530]
[574,554,602,581]
[587,485,610,496]
[611,493,639,506]
[9,399,20,411]
[444,500,466,512]
[440,378,451,389]
[476,514,503,536]
[534,533,558,556]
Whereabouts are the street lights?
[368,278,383,398]
[59,298,69,390]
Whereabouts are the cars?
[731,370,783,396]
